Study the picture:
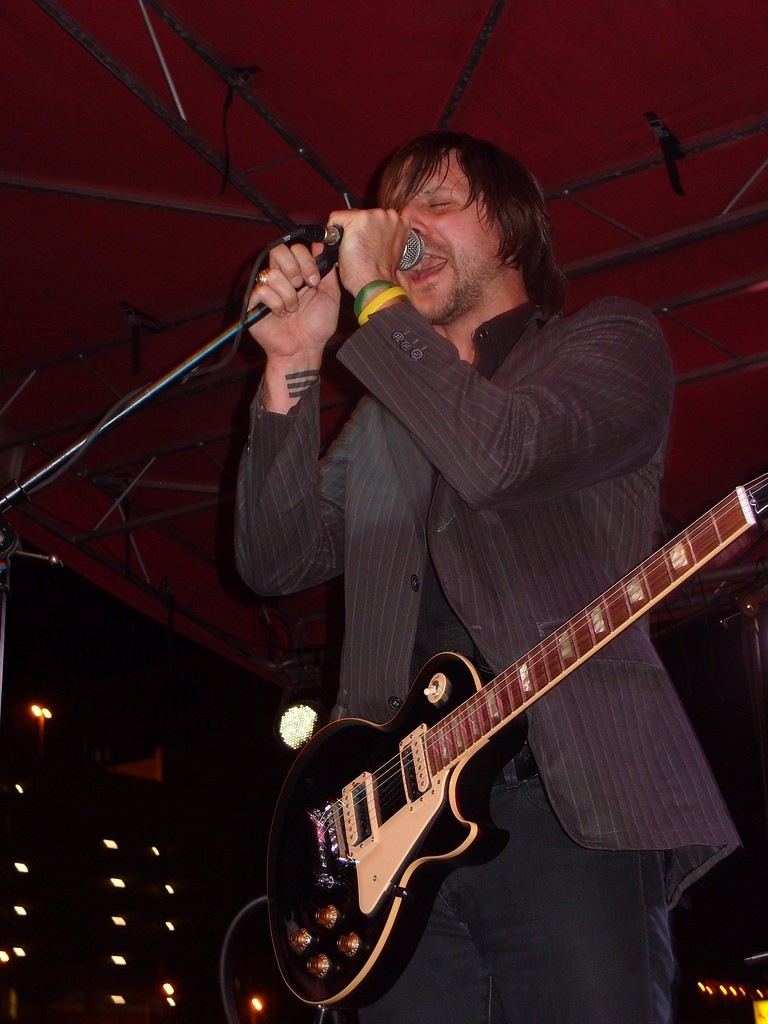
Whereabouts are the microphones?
[294,223,424,271]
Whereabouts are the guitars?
[267,465,768,1012]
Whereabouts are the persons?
[232,122,739,1024]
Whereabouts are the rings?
[255,267,271,285]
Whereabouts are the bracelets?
[357,286,409,326]
[354,279,394,318]
